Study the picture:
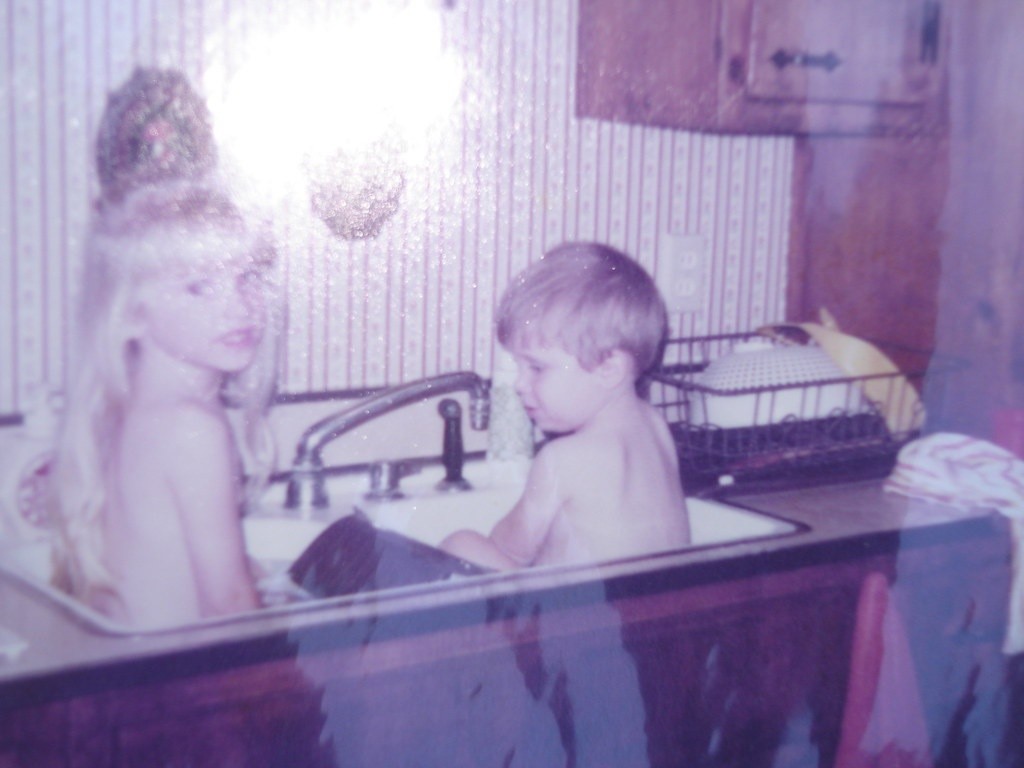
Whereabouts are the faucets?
[286,368,492,512]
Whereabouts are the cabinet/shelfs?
[577,1,949,139]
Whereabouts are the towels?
[834,570,931,768]
[883,428,1023,656]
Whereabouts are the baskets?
[641,326,949,496]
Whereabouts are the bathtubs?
[4,473,813,641]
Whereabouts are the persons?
[437,241,690,571]
[50,169,276,626]
